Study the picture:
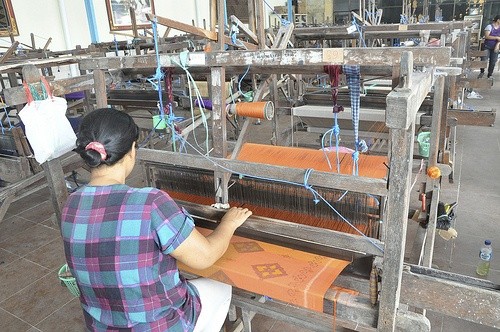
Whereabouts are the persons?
[476,14,500,86]
[59,108,253,332]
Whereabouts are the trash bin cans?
[57,262,81,297]
[416,131,431,158]
[243,91,254,102]
[151,114,168,130]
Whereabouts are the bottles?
[475,240,493,275]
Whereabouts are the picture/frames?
[105,0,155,31]
[0,0,19,38]
